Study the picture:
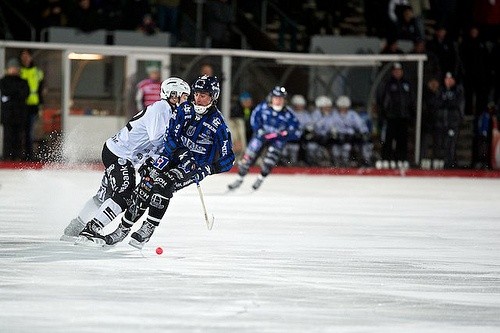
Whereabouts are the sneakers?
[252,179,263,190]
[129,221,156,249]
[60,218,106,247]
[104,223,131,247]
[227,180,242,192]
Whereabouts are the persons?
[102,74,235,252]
[228,85,302,191]
[60,77,190,247]
[0,0,180,43]
[0,49,47,162]
[135,65,161,111]
[174,0,500,171]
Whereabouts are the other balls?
[155,247,164,255]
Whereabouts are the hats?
[8,58,19,67]
[392,62,403,70]
[241,91,250,100]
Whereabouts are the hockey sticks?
[197,181,216,231]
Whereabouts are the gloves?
[179,150,212,183]
[256,129,287,146]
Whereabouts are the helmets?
[292,94,305,105]
[146,65,160,74]
[337,96,351,107]
[191,75,220,99]
[315,96,332,107]
[160,77,191,99]
[272,87,287,98]
